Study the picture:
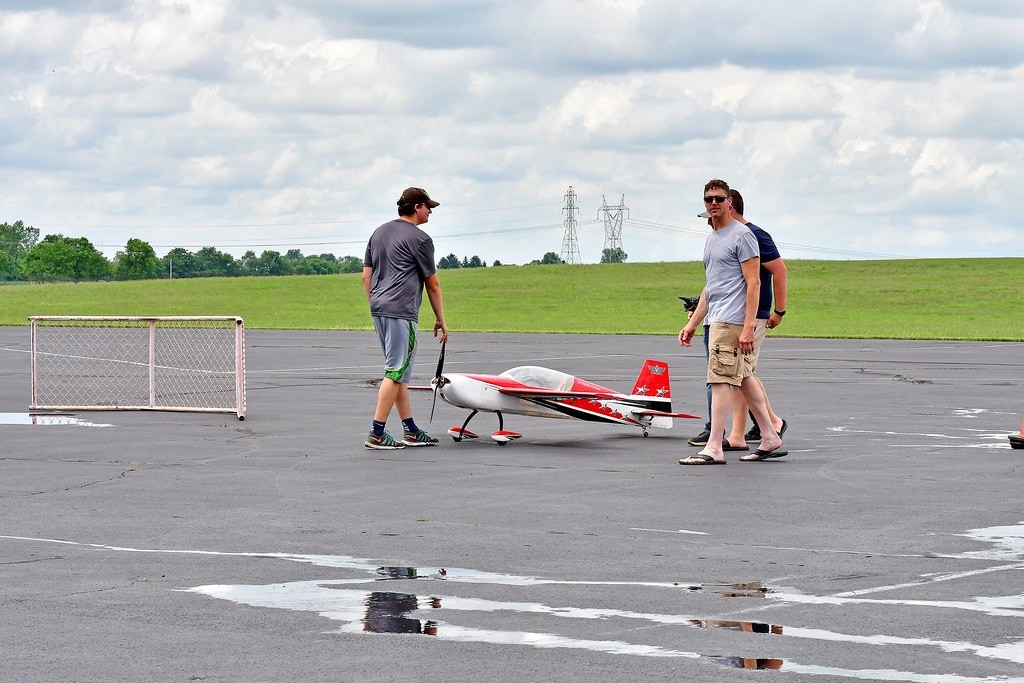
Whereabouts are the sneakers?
[687,431,724,446]
[401,429,439,446]
[745,423,763,444]
[364,429,405,450]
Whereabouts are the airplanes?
[405,338,701,446]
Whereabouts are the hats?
[698,211,712,218]
[397,186,439,208]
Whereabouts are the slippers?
[679,453,726,465]
[740,449,788,461]
[721,439,750,450]
[759,419,788,445]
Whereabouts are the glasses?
[704,194,728,203]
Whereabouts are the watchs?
[774,309,786,316]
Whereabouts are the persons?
[362,187,448,450]
[678,179,788,465]
